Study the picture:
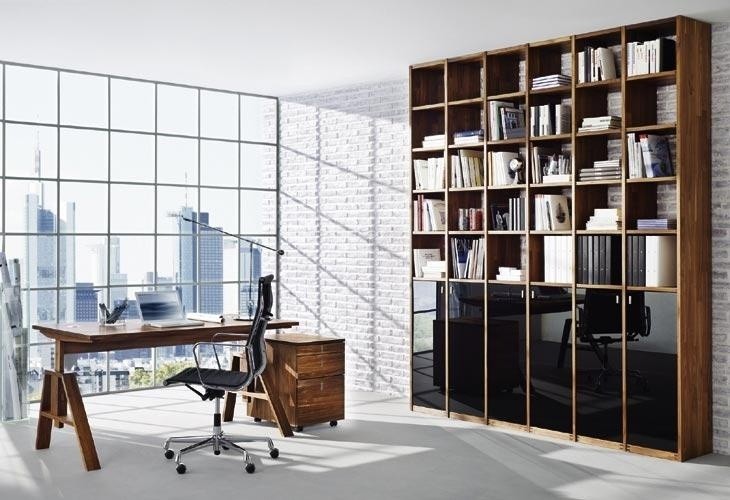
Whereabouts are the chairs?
[162,273,279,475]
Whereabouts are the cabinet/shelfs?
[246,333,344,432]
[409,16,713,463]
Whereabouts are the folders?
[627,237,644,286]
[576,235,620,286]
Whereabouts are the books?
[414,100,525,282]
[519,37,677,92]
[531,85,678,287]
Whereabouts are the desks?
[32,314,299,472]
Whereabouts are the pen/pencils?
[98,303,126,324]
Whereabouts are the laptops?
[135,290,205,328]
